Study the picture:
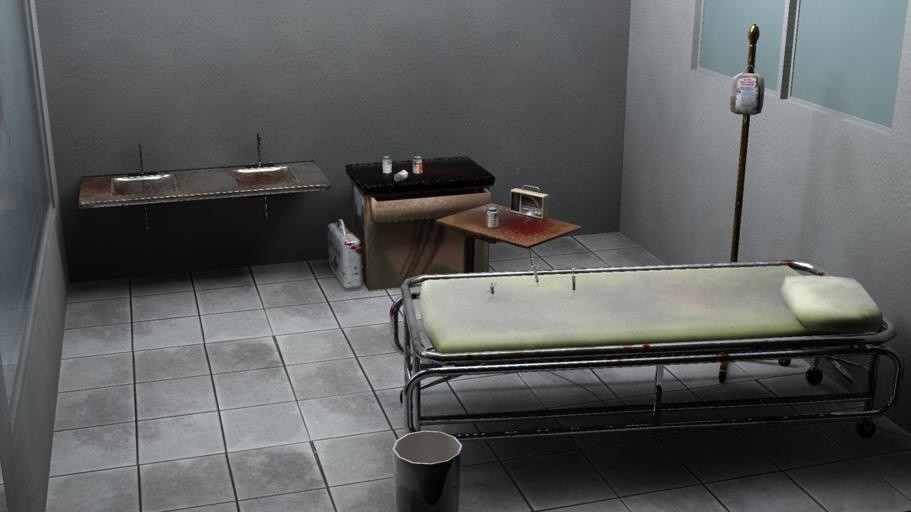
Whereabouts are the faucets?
[137,142,145,173]
[256,131,264,166]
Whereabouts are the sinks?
[230,163,301,189]
[110,173,179,195]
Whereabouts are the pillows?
[780,275,884,332]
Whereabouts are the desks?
[435,202,583,272]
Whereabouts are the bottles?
[382,155,393,175]
[486,207,500,229]
[393,169,409,182]
[412,154,423,174]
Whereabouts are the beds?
[388,260,901,440]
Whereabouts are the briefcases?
[324,218,363,289]
